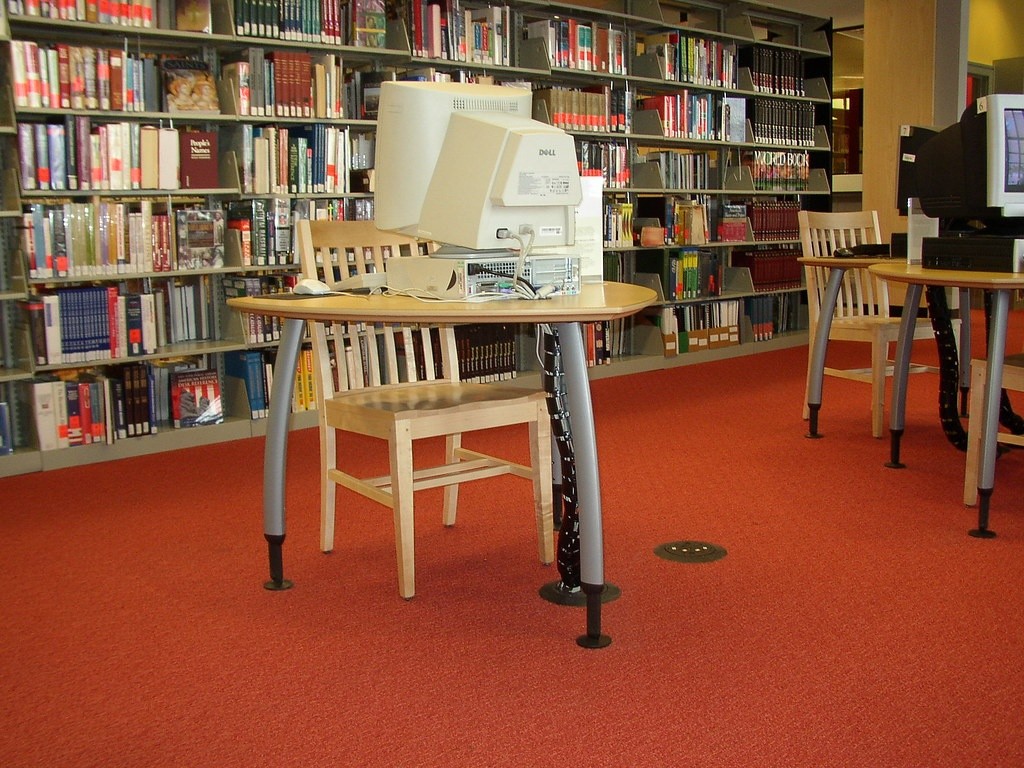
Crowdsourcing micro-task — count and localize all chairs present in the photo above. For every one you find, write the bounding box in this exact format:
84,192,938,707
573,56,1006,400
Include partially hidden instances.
296,215,558,600
962,352,1024,506
797,208,963,440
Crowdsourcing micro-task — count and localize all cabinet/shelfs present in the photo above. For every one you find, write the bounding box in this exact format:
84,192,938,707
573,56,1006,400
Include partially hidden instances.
520,0,665,382
0,0,252,472
0,1,42,478
627,1,832,376
217,0,552,442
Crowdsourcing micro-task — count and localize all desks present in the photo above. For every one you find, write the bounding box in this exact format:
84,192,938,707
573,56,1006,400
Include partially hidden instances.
867,263,1024,538
796,255,973,469
227,277,658,648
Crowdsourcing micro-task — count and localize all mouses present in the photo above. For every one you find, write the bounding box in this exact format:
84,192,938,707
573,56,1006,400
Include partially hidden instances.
293,279,330,295
834,247,854,258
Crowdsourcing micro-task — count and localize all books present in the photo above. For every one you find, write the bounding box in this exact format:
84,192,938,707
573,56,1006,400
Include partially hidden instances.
0,1,834,460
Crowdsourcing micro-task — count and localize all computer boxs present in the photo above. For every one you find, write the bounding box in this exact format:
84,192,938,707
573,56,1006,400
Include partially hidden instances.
921,236,1024,272
385,256,582,302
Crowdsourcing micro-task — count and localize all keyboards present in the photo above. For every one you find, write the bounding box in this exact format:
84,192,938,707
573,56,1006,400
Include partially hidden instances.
333,272,388,295
852,243,890,259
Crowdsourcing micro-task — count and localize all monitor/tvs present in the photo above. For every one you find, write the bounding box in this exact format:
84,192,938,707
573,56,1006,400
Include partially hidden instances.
896,125,942,217
914,94,1024,236
373,81,582,260
